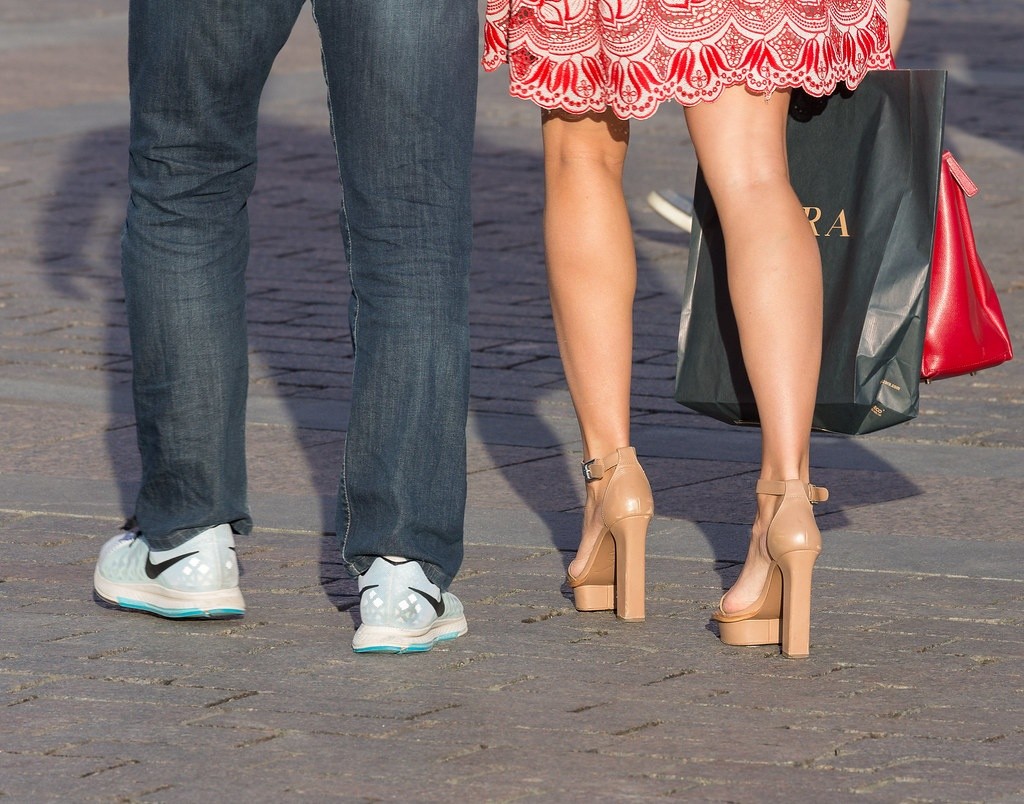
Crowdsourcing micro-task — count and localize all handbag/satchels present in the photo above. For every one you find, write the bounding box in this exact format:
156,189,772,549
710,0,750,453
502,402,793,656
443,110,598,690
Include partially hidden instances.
674,68,949,436
921,148,1014,385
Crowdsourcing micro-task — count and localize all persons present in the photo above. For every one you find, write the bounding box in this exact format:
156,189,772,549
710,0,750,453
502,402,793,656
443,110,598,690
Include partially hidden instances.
93,1,478,652
482,1,911,657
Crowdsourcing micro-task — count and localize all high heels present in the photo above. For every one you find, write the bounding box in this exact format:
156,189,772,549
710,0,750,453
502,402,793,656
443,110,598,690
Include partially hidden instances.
715,478,828,659
566,446,653,620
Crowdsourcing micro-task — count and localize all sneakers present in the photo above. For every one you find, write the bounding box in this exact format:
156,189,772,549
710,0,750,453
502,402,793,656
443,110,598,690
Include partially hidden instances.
94,522,245,618
351,554,468,654
